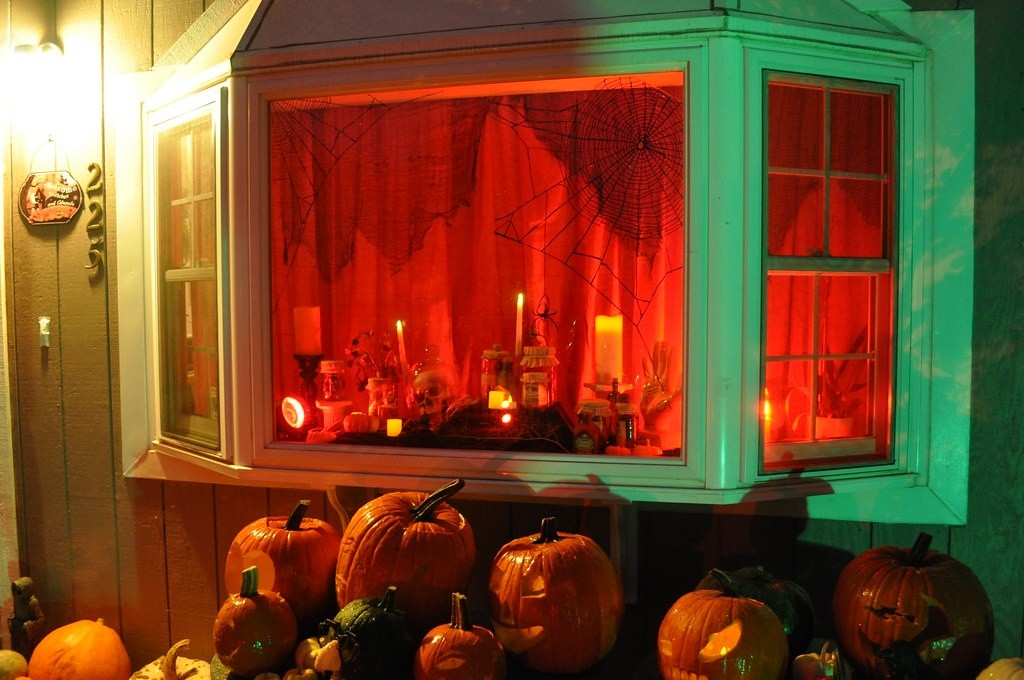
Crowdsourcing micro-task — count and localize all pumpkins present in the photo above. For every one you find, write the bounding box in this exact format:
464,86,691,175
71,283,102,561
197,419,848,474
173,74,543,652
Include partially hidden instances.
973,657,1024,680
0,617,211,680
254,635,333,680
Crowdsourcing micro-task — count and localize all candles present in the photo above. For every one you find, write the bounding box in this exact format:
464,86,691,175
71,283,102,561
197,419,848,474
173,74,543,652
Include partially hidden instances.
294,306,322,355
387,419,402,436
595,314,623,385
515,294,524,353
397,322,407,368
488,389,505,406
